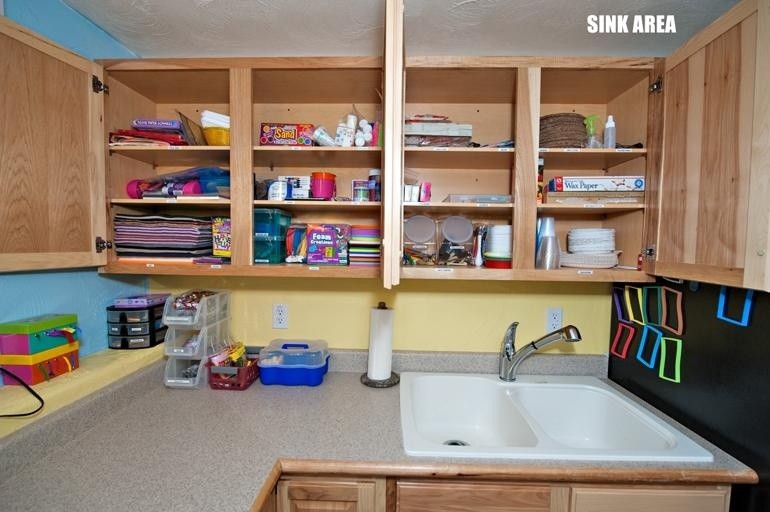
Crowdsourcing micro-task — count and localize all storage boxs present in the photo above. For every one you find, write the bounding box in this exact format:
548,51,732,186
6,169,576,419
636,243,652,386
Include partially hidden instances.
106,291,331,390
252,205,293,263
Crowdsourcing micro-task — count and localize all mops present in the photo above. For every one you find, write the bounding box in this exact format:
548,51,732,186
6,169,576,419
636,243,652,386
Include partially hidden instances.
366,307,396,381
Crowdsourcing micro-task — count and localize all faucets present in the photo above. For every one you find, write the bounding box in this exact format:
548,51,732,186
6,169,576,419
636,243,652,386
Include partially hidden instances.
498,322,583,384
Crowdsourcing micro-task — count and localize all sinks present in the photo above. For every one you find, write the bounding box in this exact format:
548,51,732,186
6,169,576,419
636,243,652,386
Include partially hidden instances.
513,373,716,462
399,371,538,458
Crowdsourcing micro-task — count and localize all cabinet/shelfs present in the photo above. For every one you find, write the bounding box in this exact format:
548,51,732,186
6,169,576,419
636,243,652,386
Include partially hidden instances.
277,475,393,511
392,477,734,512
1,1,770,293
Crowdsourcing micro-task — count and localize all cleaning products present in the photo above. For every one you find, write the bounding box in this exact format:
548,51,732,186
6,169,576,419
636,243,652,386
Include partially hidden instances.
583,113,606,149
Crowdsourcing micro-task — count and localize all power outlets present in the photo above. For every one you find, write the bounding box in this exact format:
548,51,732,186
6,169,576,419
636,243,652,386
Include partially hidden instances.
546,306,563,335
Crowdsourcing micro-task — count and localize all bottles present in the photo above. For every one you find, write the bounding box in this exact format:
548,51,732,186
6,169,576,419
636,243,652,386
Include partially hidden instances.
603,111,618,152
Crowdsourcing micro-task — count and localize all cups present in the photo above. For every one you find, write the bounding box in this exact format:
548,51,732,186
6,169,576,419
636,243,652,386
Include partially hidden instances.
308,171,337,200
